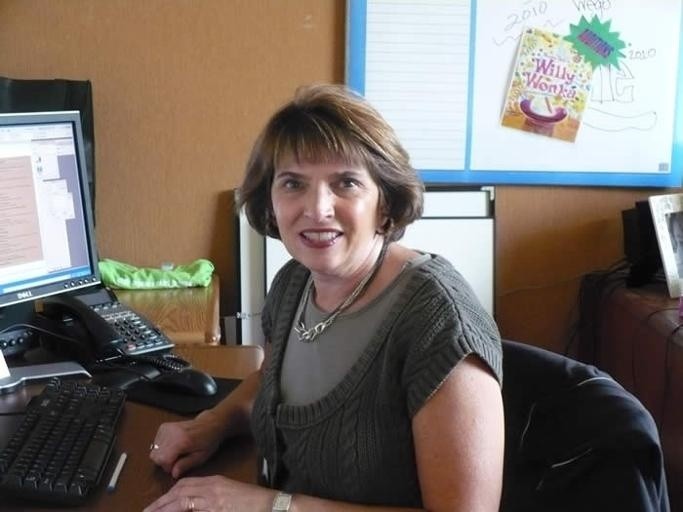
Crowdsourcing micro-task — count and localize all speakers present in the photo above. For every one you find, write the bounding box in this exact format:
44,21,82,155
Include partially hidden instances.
0,300,40,358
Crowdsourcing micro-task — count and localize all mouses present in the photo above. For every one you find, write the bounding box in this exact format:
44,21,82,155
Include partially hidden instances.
150,367,218,396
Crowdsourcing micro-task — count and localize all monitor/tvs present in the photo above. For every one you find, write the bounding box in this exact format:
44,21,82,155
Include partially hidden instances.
0,110,102,389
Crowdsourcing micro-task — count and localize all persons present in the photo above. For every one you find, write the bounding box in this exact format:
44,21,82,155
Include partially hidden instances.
142,83,506,512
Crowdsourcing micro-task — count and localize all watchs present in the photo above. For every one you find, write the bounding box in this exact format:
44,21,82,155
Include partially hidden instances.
272,491,295,511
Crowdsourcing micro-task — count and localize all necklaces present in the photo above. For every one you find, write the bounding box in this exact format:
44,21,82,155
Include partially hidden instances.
294,245,387,342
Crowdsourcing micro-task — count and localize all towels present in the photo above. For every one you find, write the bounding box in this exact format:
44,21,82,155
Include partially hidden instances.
97,254,215,290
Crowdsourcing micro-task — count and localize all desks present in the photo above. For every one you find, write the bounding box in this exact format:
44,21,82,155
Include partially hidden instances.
2,346,268,512
583,271,683,511
110,272,222,345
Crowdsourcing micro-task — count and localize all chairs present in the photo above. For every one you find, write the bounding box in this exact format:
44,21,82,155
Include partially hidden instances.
499,337,670,511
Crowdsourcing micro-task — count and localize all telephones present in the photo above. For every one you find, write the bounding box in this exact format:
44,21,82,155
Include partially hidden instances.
40,284,179,367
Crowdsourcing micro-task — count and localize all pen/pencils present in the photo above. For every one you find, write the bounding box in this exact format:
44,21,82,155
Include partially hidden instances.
108,452,128,491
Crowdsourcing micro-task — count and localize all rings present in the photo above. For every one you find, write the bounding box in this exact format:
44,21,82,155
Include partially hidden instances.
187,496,197,511
148,443,159,450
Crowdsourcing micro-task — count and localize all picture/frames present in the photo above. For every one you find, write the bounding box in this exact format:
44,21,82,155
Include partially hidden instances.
646,193,683,299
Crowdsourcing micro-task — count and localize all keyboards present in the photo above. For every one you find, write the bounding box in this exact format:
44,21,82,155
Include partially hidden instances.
0,377,128,507
